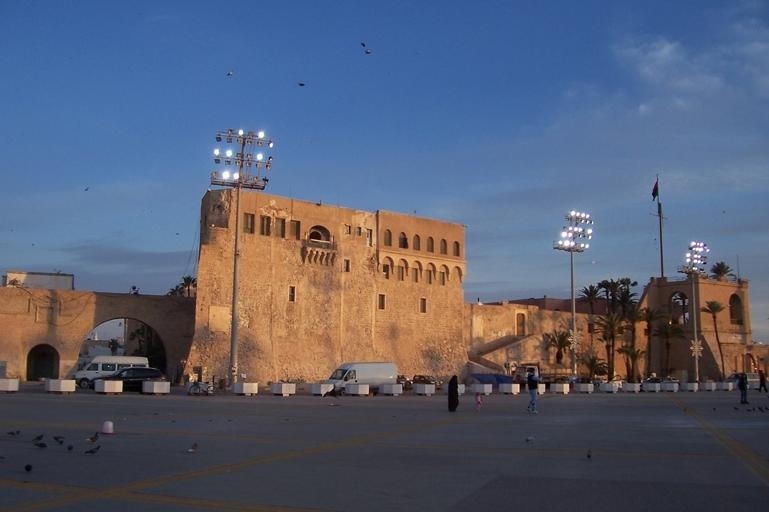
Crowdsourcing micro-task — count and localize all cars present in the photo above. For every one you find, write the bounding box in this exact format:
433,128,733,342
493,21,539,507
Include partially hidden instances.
641,376,681,384
568,375,602,384
87,368,166,391
725,372,761,390
398,374,443,391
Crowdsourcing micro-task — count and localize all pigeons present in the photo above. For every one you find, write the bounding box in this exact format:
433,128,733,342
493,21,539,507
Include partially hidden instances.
30,431,102,456
587,448,593,459
7,429,21,437
186,441,198,453
526,434,537,443
25,464,33,472
712,405,769,414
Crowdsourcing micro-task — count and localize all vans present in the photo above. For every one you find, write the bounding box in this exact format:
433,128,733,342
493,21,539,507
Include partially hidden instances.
326,361,398,396
72,355,150,389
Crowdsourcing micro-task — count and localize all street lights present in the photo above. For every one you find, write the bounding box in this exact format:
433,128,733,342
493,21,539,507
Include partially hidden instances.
211,126,274,393
677,241,711,384
176,359,187,383
552,209,594,378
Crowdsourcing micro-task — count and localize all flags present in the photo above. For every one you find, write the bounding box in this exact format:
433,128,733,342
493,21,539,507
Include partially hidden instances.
652,181,658,201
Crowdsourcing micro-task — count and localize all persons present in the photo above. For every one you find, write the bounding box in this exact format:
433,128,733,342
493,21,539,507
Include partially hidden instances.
525,369,543,415
448,374,459,411
757,369,767,393
739,371,749,405
475,391,484,410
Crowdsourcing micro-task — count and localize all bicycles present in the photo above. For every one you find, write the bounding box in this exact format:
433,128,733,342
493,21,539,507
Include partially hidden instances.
189,380,217,396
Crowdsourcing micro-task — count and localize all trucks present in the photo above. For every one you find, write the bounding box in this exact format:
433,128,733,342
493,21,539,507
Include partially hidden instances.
512,362,572,385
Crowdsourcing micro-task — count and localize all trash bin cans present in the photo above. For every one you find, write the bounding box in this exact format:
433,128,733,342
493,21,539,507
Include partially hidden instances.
219,378,225,389
